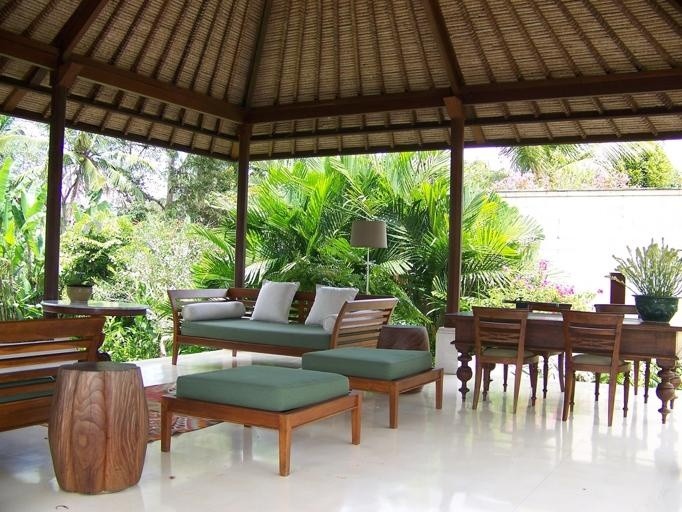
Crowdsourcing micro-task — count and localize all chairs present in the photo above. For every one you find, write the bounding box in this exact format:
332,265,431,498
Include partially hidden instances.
595,304,651,403
558,308,632,425
0,317,108,431
471,305,539,414
503,301,572,398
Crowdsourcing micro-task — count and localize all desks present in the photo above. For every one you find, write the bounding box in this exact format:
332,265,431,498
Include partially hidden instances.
39,300,147,362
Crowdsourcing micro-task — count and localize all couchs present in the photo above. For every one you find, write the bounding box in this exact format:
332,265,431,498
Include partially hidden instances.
167,288,399,365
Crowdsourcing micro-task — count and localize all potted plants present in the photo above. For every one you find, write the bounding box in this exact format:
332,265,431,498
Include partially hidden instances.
62,255,101,304
604,236,682,323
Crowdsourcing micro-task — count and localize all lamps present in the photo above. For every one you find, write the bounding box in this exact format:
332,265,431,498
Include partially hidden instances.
350,221,388,292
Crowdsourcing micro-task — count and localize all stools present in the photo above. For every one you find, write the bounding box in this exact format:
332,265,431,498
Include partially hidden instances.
161,364,362,475
302,347,444,429
48,361,149,495
377,325,430,395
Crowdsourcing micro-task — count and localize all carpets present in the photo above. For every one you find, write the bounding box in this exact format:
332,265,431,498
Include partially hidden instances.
41,382,224,443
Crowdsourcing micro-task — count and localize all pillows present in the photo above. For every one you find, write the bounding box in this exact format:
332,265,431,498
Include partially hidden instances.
304,284,359,326
250,279,301,324
181,301,245,321
322,309,374,335
